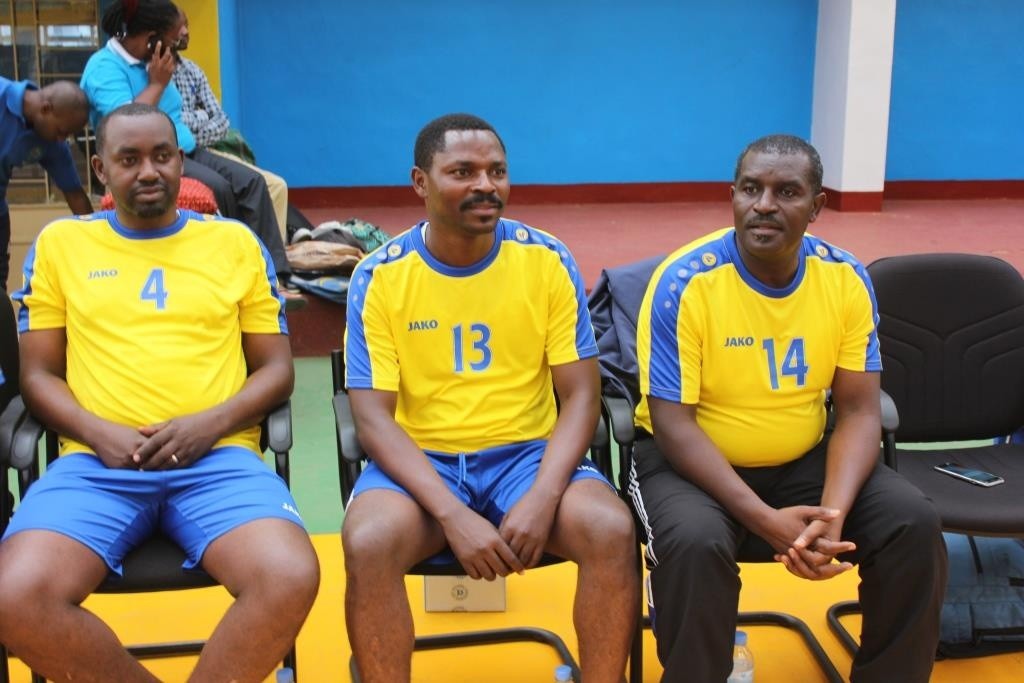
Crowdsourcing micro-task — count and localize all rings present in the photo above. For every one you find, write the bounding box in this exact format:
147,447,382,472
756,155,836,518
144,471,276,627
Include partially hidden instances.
172,454,179,464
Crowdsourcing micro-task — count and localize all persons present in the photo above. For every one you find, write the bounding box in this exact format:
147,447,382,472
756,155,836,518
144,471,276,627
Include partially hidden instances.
0,76,93,393
0,105,321,683
343,114,639,682
626,133,950,683
80,0,308,312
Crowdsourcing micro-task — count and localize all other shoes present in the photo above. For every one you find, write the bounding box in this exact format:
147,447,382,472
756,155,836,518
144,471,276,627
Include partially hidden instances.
280,289,308,310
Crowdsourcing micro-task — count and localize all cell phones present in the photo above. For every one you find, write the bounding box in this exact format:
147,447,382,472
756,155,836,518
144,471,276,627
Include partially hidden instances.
933,461,1005,487
149,32,164,57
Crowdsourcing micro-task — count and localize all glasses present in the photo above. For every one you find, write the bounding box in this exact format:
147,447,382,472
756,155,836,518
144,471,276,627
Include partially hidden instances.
160,35,181,47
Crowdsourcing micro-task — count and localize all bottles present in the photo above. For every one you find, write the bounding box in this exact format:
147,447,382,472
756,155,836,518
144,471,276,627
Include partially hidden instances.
727,631,754,683
276,668,294,683
555,665,572,683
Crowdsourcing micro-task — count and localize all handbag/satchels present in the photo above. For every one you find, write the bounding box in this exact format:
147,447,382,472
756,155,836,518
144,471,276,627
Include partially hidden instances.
284,216,395,275
935,532,1024,658
218,130,256,164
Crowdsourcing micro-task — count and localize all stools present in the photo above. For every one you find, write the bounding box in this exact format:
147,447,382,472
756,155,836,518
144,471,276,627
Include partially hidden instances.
99,176,218,214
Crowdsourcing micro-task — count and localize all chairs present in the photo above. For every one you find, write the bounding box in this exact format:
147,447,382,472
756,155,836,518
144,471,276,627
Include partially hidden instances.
329,325,616,683
599,253,844,683
826,253,1024,659
0,399,298,683
